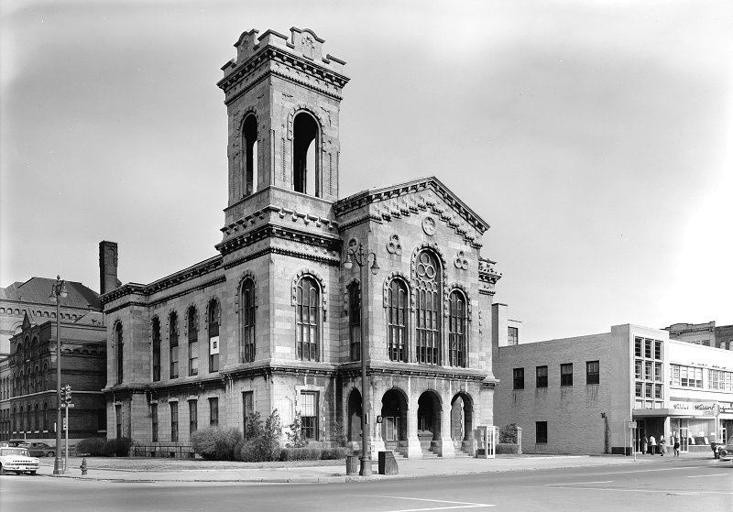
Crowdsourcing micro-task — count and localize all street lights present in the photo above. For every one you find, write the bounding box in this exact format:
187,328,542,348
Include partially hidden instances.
48,275,68,474
343,242,380,476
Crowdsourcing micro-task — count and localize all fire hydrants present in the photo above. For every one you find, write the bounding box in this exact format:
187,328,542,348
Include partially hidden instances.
80,458,87,475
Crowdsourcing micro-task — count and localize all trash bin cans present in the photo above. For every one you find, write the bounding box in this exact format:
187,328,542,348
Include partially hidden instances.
346,456,359,475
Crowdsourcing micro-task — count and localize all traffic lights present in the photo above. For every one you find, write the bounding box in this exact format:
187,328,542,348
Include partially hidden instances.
61,386,72,402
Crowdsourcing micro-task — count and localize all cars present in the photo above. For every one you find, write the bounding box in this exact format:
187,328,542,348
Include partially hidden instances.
0,441,56,476
720,436,733,461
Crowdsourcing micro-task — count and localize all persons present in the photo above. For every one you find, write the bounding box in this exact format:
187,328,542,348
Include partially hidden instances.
642,433,649,455
649,433,657,456
658,434,667,456
672,436,680,457
